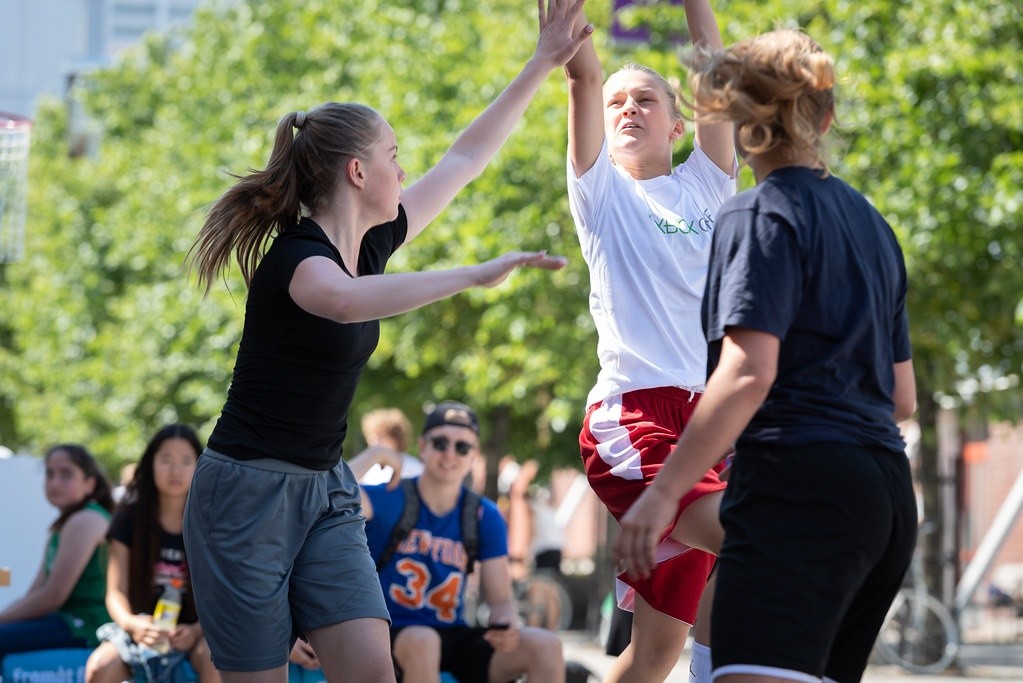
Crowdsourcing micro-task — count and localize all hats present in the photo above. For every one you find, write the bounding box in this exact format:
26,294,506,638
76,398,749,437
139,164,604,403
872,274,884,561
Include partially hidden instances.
423,400,479,436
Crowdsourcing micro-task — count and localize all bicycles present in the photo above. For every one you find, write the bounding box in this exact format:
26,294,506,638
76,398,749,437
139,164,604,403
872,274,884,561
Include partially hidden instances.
874,522,959,676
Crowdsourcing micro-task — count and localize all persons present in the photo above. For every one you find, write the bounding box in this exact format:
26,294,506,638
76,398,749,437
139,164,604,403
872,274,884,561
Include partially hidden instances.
0,400,570,683
611,30,916,682
182,0,594,682
558,1,739,683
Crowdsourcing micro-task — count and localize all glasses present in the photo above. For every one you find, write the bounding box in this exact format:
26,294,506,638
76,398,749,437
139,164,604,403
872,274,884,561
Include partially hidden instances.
429,436,477,456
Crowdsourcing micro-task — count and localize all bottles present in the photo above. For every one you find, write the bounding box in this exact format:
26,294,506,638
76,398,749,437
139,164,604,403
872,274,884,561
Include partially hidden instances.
153,578,183,637
